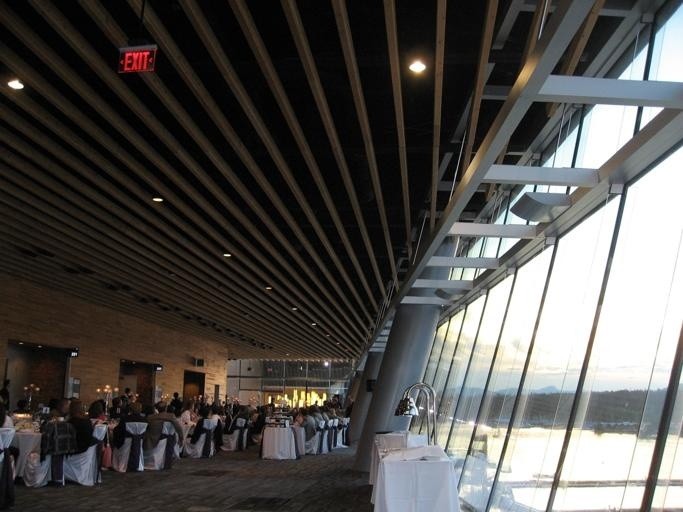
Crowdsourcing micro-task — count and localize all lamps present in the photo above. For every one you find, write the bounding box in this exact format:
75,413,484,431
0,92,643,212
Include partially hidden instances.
400,383,437,446
394,387,430,446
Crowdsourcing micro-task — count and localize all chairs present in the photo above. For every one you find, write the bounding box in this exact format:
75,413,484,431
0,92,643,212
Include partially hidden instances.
251,418,350,461
101,417,252,474
1,419,107,490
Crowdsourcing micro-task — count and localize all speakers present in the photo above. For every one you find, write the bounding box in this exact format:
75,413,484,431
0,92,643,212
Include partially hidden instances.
197,358,204,366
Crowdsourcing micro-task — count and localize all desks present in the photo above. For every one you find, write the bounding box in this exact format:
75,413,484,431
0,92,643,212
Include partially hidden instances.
370,433,460,512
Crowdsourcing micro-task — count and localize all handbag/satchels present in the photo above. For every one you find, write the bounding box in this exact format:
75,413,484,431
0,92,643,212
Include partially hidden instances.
101,445,111,468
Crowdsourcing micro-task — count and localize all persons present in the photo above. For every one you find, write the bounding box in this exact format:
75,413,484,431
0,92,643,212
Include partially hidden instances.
192,393,354,452
1,378,198,472
